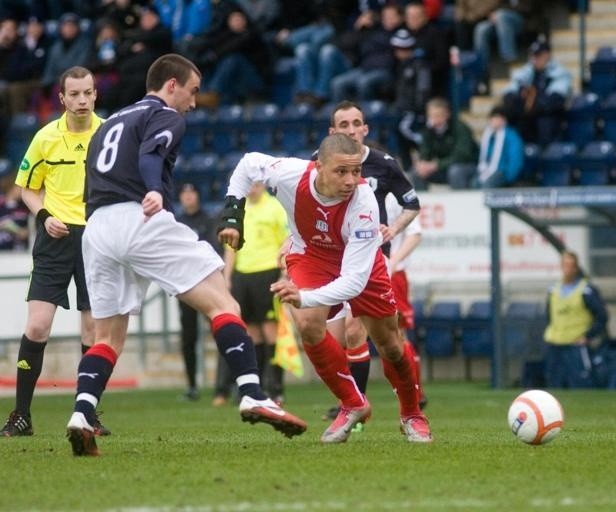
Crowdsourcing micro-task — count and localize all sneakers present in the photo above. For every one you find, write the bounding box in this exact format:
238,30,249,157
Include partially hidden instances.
419,391,428,409
399,411,434,444
320,391,371,443
238,390,308,439
0,410,34,438
176,388,286,407
65,412,111,457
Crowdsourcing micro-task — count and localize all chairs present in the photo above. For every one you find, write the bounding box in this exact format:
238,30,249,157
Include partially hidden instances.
366,299,547,383
521,49,615,186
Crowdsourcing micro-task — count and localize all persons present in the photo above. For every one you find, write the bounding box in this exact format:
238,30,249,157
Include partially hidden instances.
230,181,292,410
1,0,575,189
171,179,229,410
216,133,432,446
63,53,308,456
309,98,428,421
542,251,608,387
0,67,111,440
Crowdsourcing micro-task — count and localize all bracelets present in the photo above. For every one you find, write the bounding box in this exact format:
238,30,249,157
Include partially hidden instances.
35,208,52,227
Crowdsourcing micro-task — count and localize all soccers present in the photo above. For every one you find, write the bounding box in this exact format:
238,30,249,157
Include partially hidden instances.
508,390,563,444
